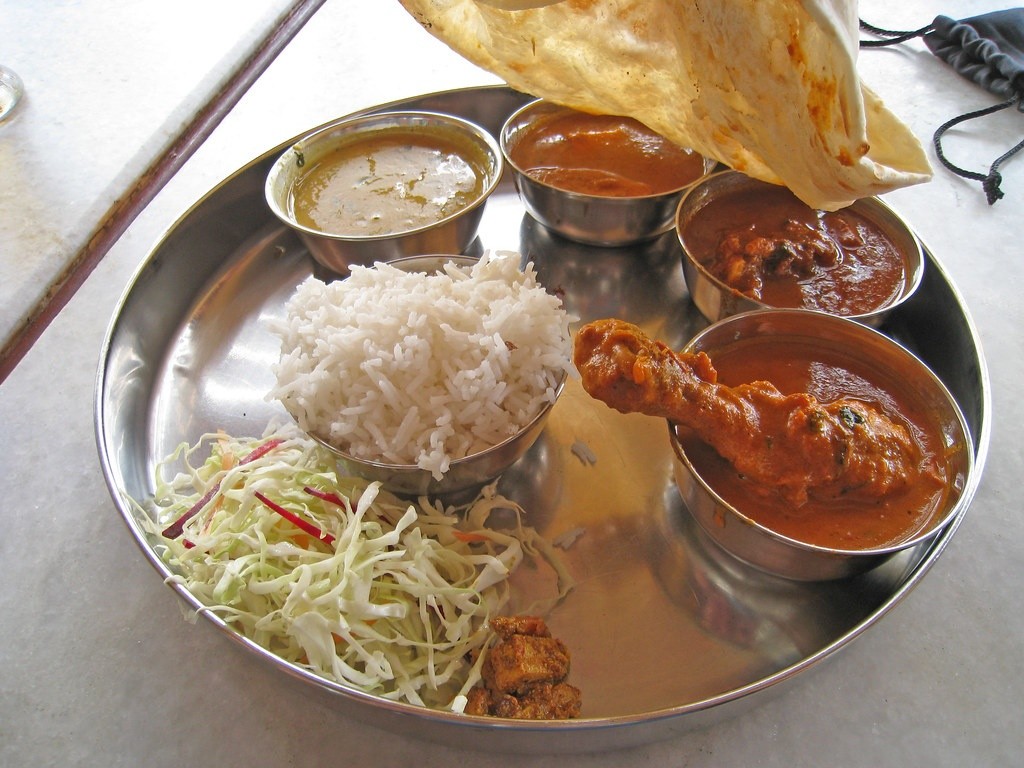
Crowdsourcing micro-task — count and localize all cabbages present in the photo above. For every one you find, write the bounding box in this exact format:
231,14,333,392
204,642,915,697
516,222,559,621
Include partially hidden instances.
117,422,576,715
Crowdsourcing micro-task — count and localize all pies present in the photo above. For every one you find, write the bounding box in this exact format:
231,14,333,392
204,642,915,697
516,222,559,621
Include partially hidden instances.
402,0,935,211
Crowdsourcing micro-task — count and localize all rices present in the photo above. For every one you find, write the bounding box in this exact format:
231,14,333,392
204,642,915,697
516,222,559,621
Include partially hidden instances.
263,250,597,552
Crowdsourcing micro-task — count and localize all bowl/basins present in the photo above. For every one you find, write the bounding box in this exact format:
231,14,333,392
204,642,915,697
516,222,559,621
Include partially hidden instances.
265,111,504,276
666,308,976,581
499,98,718,247
279,253,572,495
674,169,925,333
